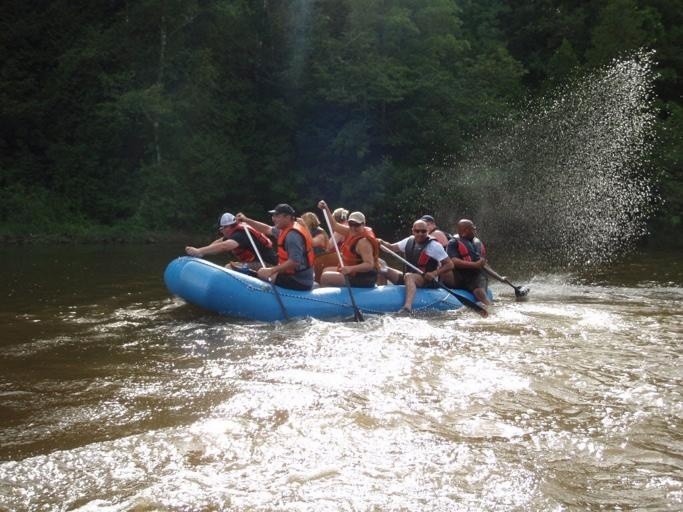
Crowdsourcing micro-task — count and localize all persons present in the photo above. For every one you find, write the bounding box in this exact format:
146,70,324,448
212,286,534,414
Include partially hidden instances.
316,200,376,288
183,212,277,269
295,210,329,256
375,219,455,315
312,206,351,280
420,214,449,251
233,202,313,292
437,218,491,306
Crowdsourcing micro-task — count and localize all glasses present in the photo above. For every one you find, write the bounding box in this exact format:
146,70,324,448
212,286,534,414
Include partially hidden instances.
348,223,364,228
413,228,428,234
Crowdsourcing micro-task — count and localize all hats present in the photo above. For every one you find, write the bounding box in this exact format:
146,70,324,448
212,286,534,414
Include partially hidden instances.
420,214,435,223
216,211,237,228
346,210,367,226
266,202,297,217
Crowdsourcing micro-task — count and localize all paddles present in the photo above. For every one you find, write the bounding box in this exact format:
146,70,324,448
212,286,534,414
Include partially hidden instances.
321,208,366,322
504,278,529,298
379,243,488,320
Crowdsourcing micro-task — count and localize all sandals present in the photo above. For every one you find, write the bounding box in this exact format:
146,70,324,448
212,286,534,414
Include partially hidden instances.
396,306,413,318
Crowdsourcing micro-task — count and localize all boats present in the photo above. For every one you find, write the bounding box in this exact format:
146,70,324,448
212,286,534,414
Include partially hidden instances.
161,254,494,325
